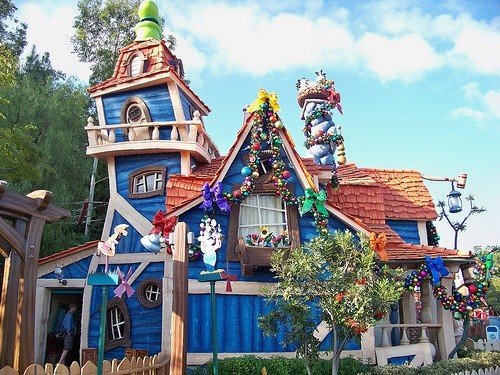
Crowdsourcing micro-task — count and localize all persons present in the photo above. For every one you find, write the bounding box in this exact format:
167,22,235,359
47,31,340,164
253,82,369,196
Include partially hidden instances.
55,302,79,365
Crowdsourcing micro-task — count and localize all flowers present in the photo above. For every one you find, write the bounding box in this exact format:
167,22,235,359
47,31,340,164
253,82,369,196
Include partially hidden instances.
245,226,290,248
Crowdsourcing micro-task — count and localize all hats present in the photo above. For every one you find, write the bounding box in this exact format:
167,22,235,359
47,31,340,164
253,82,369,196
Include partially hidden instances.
68,303,78,308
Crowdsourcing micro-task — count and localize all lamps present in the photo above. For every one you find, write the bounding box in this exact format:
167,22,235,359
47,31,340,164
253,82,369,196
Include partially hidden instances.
447,180,462,213
54,264,67,286
140,233,175,253
